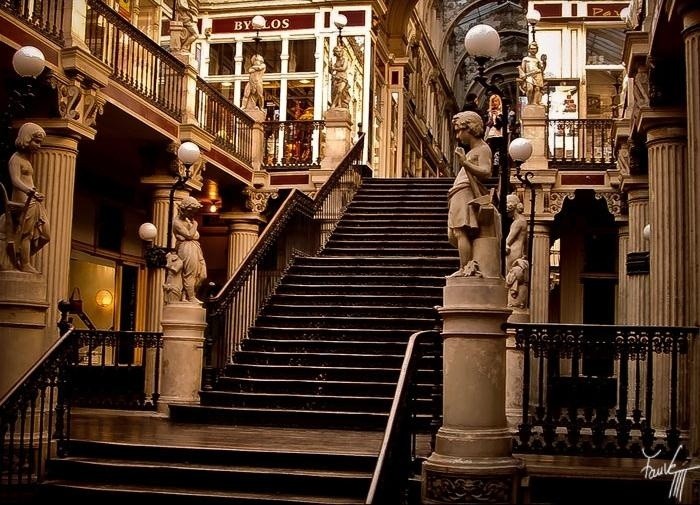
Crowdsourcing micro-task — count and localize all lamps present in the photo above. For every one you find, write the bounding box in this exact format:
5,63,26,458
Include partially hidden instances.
138,142,220,269
334,14,348,46
641,224,650,265
0,46,45,201
252,15,266,57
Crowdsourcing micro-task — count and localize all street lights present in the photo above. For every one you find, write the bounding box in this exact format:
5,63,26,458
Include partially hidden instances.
248,16,264,57
335,14,347,48
5,46,45,118
138,141,201,270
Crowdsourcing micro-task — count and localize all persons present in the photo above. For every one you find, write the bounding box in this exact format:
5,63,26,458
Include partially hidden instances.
328,46,349,109
483,92,508,177
462,93,478,111
522,41,543,104
172,196,208,304
8,123,51,274
445,110,492,277
175,0,199,52
240,54,267,113
506,194,528,276
